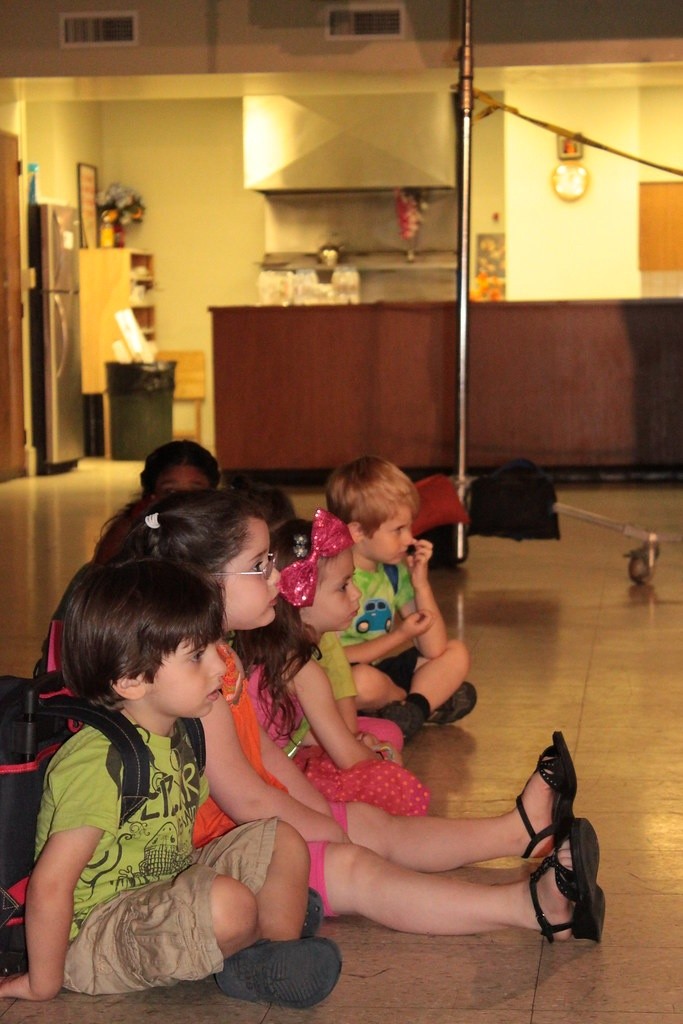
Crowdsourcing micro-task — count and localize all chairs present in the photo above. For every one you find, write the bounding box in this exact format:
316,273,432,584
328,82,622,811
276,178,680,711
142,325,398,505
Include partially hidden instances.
158,351,206,443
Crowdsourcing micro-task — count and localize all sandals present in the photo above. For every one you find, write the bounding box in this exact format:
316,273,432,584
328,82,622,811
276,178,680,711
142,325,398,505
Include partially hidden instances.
300,886,326,941
516,731,576,859
529,818,604,943
214,935,343,1009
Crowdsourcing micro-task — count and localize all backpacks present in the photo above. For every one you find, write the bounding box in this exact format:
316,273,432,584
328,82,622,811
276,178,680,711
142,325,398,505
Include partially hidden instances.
0,671,206,977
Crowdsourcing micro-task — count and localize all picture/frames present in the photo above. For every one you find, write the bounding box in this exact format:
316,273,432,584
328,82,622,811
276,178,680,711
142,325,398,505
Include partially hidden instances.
559,133,584,160
77,162,100,249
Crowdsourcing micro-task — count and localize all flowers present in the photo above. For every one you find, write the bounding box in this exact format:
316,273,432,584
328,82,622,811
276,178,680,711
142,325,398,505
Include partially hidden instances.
94,179,146,226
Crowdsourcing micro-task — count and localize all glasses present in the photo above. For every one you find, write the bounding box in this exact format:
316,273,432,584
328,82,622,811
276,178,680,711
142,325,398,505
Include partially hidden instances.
207,549,279,580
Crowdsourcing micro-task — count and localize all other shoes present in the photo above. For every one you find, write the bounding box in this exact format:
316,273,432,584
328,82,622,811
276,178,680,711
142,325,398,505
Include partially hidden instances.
426,681,477,724
374,700,423,740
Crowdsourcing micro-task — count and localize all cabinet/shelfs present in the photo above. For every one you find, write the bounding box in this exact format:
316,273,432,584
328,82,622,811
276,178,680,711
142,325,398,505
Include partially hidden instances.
78,250,154,395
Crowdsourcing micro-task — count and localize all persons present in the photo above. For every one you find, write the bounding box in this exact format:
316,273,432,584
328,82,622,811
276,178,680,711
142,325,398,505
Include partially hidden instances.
0,557,341,1007
102,438,605,942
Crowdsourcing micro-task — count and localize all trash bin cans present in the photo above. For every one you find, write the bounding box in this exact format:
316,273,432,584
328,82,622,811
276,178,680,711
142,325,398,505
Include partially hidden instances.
105,361,177,460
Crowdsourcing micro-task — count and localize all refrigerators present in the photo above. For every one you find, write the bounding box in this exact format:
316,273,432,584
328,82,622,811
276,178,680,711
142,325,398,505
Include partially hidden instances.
25,200,84,477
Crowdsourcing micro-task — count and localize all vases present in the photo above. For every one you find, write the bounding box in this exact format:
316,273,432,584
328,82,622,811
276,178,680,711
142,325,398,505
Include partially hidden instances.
112,222,126,248
